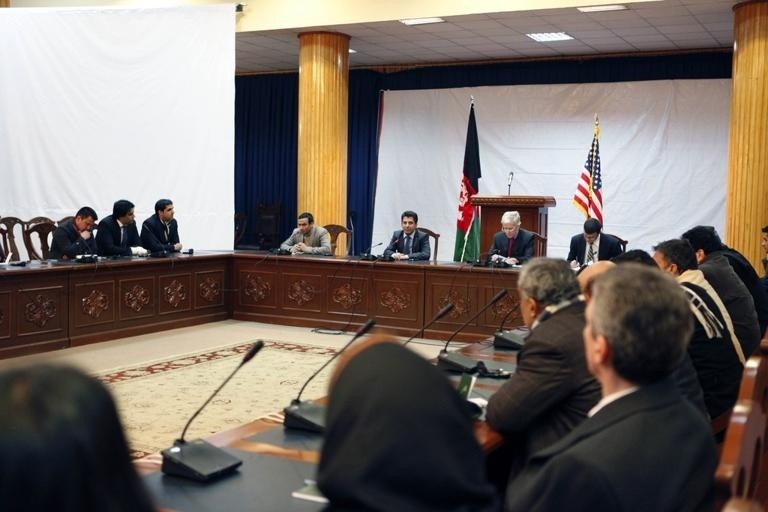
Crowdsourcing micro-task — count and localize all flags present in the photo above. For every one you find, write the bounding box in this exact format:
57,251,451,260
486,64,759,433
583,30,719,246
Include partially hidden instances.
573,132,603,230
452,103,481,262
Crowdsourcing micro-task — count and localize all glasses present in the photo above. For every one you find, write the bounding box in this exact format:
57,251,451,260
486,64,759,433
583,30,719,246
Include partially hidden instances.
501,226,515,233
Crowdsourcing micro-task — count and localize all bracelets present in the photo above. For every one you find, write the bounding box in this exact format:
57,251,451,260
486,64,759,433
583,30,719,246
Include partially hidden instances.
289,247,292,252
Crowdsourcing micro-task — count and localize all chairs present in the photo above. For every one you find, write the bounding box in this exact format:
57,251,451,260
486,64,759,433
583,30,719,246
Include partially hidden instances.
532,232,547,257
611,235,628,252
0,216,97,261
418,227,440,260
322,224,352,256
715,330,768,512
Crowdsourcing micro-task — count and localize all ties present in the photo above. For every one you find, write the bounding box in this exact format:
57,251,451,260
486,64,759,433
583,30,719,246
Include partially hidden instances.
121,225,129,247
403,235,411,256
507,238,515,256
587,242,594,262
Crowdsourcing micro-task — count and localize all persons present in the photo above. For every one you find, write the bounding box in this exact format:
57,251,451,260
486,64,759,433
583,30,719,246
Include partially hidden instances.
316,334,495,512
280,213,332,256
567,219,767,425
383,211,430,261
506,260,719,511
488,211,535,265
0,364,159,512
486,256,601,472
95,200,148,257
139,199,182,253
49,207,98,260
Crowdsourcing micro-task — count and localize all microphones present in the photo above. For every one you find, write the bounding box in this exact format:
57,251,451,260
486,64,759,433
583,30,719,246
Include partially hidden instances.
402,303,453,347
143,223,168,258
437,289,509,374
160,340,264,483
382,236,407,262
585,252,597,263
361,243,383,261
494,303,526,349
277,231,299,255
283,318,375,433
495,249,510,268
508,172,513,187
74,231,98,263
